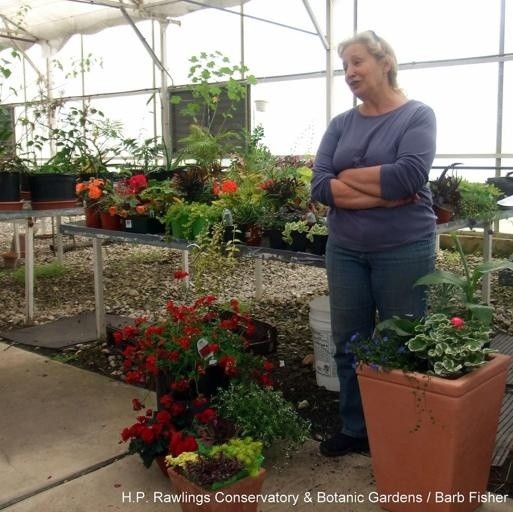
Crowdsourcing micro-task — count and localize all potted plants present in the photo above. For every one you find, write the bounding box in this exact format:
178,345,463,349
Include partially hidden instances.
163,202,191,239
261,203,284,249
145,138,190,183
305,222,328,255
163,437,269,511
244,197,261,246
30,156,66,210
61,163,81,211
213,198,243,246
97,195,121,231
209,381,312,473
115,395,223,468
16,161,36,200
280,216,309,252
0,148,24,214
430,162,462,224
75,178,104,200
74,153,114,192
149,203,167,235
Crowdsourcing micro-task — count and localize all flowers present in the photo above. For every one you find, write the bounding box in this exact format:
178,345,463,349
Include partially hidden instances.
115,172,150,196
208,175,239,198
111,271,277,390
115,199,149,217
343,234,512,380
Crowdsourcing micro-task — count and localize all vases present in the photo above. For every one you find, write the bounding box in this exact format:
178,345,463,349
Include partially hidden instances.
151,368,230,432
120,215,152,235
80,199,99,232
355,353,512,512
145,452,168,473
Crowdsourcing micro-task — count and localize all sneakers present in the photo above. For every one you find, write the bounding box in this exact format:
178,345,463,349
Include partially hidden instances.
318,431,370,462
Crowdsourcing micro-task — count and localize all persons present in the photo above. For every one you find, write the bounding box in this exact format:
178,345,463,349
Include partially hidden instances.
311,31,438,457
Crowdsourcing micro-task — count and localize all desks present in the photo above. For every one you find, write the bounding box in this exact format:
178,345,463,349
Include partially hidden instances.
0,194,200,326
54,205,513,344
